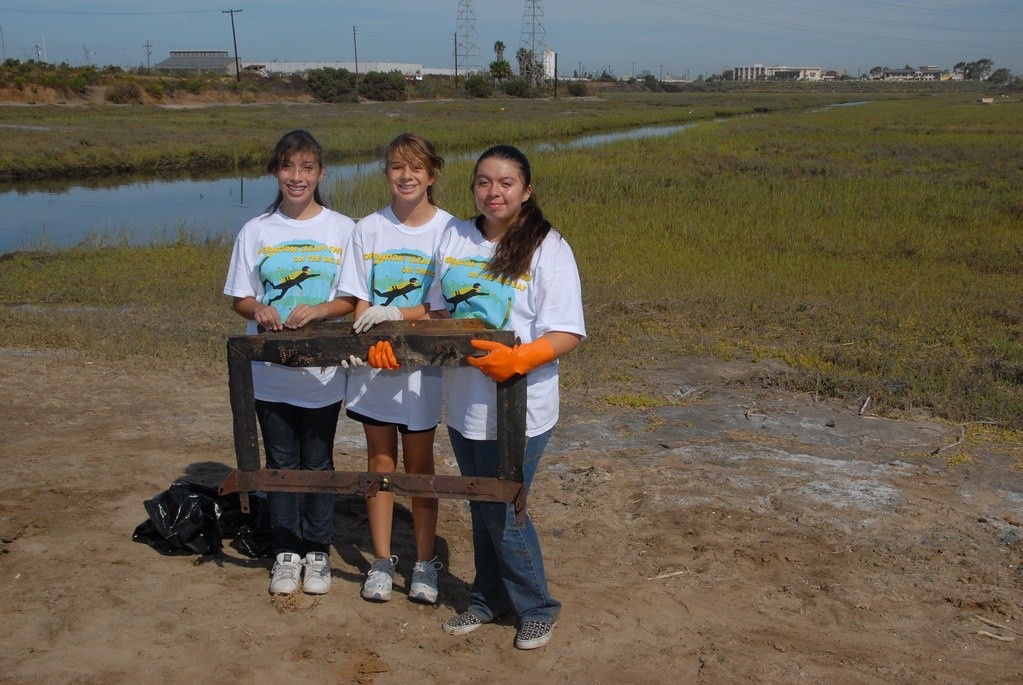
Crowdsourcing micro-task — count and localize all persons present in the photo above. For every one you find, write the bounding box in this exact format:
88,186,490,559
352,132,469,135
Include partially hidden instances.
338,136,464,603
222,129,355,595
366,143,586,648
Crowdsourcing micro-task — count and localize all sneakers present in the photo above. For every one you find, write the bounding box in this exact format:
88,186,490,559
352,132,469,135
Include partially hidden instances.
269,552,307,595
362,554,399,601
302,553,333,594
408,554,444,603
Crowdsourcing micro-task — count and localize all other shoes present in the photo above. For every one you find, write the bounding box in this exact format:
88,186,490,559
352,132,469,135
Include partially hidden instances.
515,619,558,649
442,609,483,635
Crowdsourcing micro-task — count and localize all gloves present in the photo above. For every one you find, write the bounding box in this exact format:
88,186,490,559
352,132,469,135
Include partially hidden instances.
354,304,402,335
369,339,399,372
467,337,554,383
341,356,367,375
